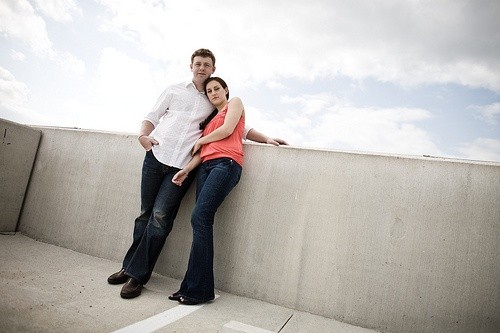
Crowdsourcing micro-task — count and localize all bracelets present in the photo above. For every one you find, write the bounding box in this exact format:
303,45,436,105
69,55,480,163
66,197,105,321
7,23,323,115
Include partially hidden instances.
138,135,144,140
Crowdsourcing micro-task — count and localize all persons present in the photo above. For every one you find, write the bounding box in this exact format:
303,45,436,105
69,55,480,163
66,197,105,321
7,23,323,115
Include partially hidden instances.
170,76,245,304
107,48,288,299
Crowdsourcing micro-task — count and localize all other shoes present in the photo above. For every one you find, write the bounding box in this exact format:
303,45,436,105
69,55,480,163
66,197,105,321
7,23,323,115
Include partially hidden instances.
168,289,215,305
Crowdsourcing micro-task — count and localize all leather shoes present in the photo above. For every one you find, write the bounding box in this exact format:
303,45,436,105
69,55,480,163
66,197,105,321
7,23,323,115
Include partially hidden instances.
120,277,144,299
108,268,129,285
